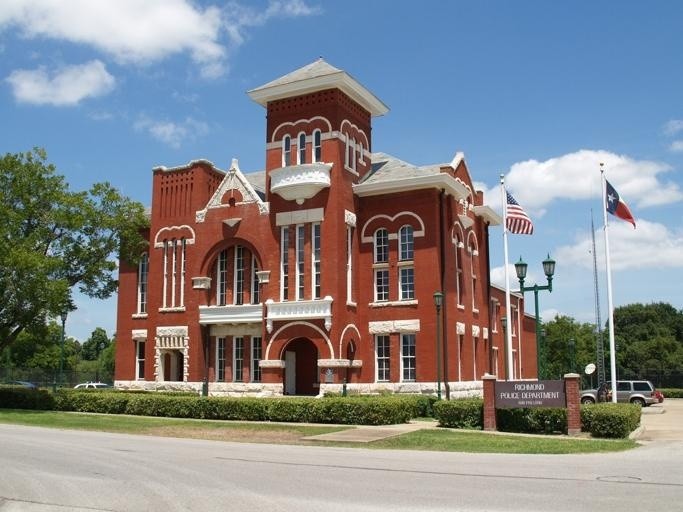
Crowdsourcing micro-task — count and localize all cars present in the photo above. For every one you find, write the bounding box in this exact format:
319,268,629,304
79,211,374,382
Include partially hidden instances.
4,380,38,391
655,389,664,402
75,382,112,389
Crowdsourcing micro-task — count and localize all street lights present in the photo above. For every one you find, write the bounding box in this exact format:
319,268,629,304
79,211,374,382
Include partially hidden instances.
499,316,509,382
95,341,105,382
430,289,443,406
515,252,559,381
56,309,70,393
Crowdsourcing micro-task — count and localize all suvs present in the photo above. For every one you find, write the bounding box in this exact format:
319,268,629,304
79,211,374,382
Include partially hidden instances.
580,379,660,407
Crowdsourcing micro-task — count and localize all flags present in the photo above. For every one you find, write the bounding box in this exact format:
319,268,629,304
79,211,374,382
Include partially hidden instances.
605,178,636,230
504,190,533,235
